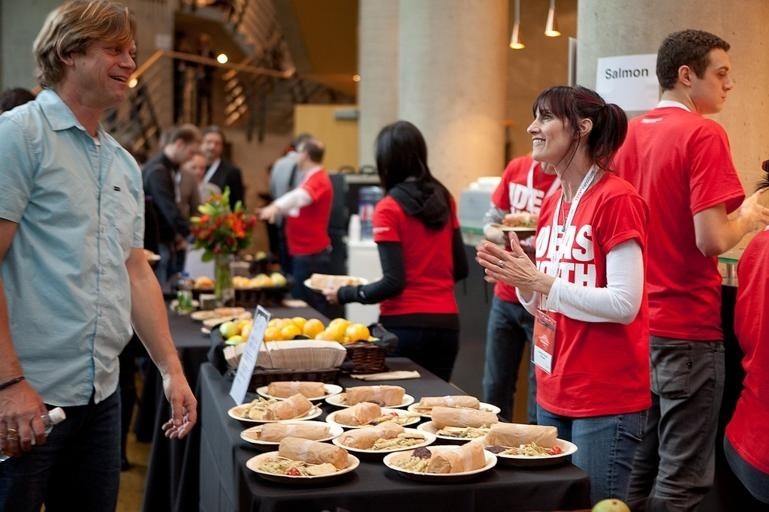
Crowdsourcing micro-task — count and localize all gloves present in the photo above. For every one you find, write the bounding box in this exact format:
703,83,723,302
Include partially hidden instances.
351,370,422,381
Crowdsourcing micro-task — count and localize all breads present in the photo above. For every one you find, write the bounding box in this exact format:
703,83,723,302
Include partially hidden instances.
277,436,350,472
347,424,410,446
345,385,407,406
262,420,328,439
269,380,326,399
306,274,360,292
267,393,315,418
430,408,498,429
421,395,481,410
485,421,559,449
433,445,485,473
335,402,384,425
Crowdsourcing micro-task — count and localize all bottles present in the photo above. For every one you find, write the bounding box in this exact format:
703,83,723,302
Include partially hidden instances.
174,271,191,316
0,407,66,463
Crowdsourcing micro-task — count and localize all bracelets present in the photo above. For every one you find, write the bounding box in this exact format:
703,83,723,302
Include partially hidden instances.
0,375,26,392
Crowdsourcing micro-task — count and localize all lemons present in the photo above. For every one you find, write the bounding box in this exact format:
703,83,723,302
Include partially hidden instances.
220,317,253,345
263,316,379,344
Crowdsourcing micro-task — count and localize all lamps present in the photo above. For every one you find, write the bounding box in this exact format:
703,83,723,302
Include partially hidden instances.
544,1,561,37
509,0,525,50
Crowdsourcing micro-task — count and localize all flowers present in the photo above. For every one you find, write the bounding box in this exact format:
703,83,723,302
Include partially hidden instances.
190,186,255,255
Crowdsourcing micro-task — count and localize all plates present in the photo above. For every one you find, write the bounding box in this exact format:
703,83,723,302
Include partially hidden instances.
226,402,323,424
417,419,488,442
499,223,537,233
255,382,343,401
244,450,360,479
240,419,343,445
381,445,498,477
325,408,419,429
325,389,414,409
471,434,578,461
407,399,501,420
305,275,368,292
331,427,436,454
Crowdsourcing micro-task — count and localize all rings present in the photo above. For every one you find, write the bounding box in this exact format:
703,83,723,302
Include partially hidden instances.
496,259,504,267
6,427,18,440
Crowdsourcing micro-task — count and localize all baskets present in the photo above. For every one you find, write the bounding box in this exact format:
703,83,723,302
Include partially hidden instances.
232,367,340,391
340,344,386,374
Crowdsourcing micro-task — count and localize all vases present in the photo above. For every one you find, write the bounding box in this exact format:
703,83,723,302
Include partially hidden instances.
214,253,233,307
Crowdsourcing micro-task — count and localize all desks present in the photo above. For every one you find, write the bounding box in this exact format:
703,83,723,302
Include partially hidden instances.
142,287,404,512
201,356,591,510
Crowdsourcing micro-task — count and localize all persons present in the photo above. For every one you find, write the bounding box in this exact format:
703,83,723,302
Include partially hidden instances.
0,86,37,113
158,127,202,223
253,138,335,309
200,130,246,214
607,26,768,512
0,0,200,511
322,119,470,385
179,151,222,206
141,123,200,291
475,84,654,511
482,153,563,427
723,158,769,512
269,132,312,278
